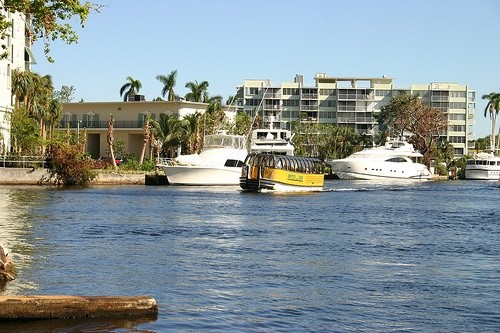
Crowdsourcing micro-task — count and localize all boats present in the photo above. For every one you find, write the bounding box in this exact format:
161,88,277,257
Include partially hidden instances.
464,152,499,180
240,152,324,192
157,115,296,186
328,135,448,181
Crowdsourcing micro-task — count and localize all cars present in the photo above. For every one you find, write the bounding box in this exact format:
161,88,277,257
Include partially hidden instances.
98,156,123,166
